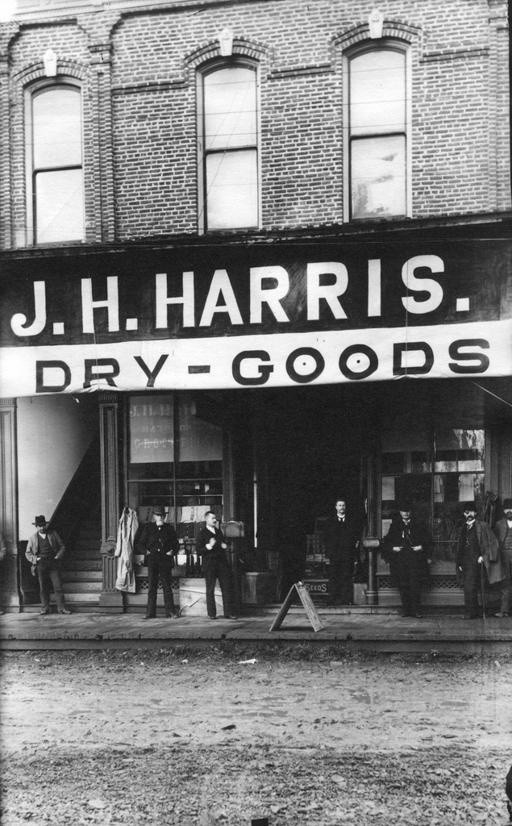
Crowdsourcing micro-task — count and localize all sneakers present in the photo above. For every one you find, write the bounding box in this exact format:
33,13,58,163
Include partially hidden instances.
142,611,178,619
40,610,48,615
58,609,72,614
208,614,239,619
459,607,512,619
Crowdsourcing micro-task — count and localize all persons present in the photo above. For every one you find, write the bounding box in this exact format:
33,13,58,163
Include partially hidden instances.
134,505,182,620
320,498,359,609
23,515,73,614
379,499,429,618
492,499,512,618
453,500,506,616
193,509,238,620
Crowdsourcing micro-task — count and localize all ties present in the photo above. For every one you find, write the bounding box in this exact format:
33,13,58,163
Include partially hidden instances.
338,517,346,528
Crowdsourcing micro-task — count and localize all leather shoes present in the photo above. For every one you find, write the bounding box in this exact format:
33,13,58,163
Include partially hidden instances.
401,608,423,619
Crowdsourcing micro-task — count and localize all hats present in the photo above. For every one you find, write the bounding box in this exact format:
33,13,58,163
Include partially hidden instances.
151,505,169,515
32,515,50,525
